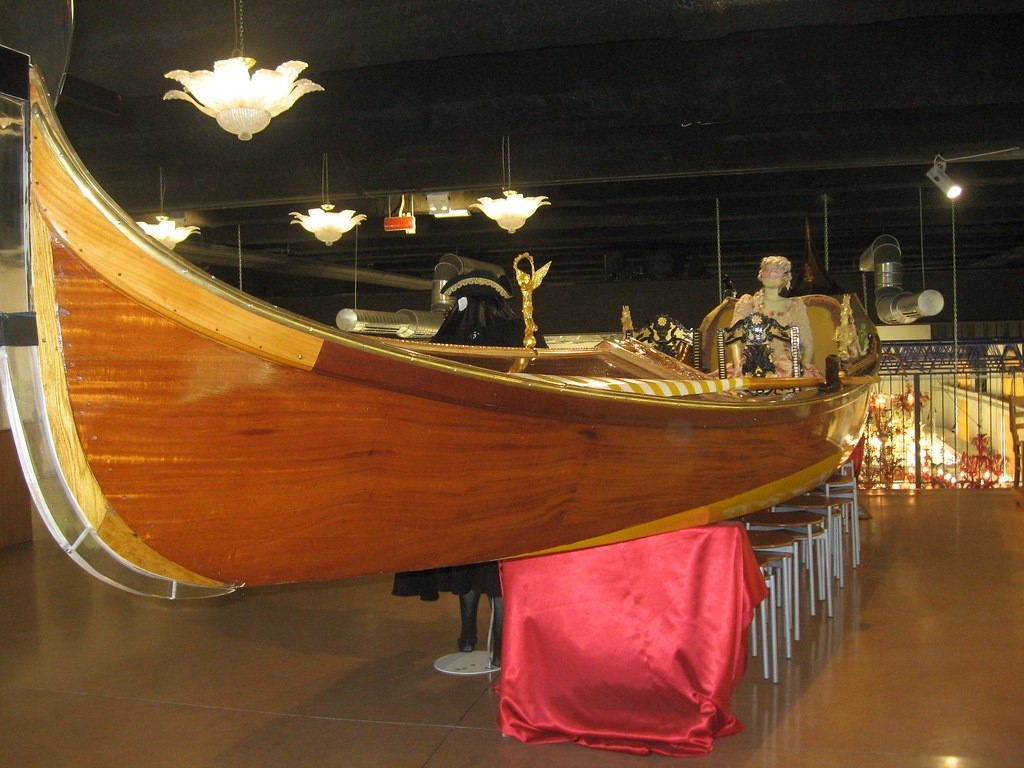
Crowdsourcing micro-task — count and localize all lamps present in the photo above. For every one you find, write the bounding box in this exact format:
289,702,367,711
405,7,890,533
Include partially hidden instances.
288,152,367,245
926,146,1020,200
162,0,327,141
468,136,553,234
135,166,202,251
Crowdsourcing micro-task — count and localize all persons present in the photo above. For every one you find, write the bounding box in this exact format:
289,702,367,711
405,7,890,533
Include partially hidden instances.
729,255,815,379
833,293,860,359
513,252,552,335
393,267,548,668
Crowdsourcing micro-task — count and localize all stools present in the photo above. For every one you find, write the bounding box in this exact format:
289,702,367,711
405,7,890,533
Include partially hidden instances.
740,460,862,684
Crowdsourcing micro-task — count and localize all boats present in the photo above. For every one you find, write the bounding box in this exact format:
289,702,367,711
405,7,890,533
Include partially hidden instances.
1,1,886,604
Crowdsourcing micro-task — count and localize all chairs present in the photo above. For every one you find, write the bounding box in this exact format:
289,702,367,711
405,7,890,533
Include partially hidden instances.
1009,395,1024,489
